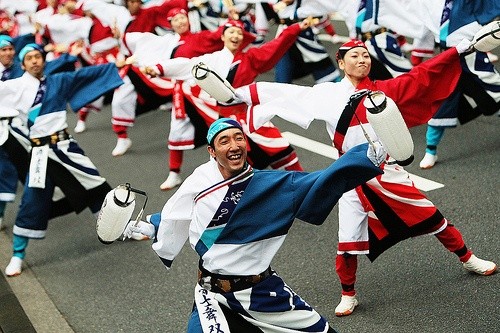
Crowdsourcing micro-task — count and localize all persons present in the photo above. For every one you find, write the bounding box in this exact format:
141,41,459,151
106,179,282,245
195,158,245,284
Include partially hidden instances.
124,120,387,333
218,39,498,316
0,0,500,275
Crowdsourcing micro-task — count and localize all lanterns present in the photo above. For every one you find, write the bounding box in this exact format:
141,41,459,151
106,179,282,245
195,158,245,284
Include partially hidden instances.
96,184,138,244
191,64,236,105
362,91,415,168
470,21,500,52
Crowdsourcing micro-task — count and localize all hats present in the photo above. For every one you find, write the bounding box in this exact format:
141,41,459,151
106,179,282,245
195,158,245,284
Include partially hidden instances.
338,41,366,57
167,8,187,23
0,34,14,49
206,118,242,144
223,21,244,32
18,43,44,61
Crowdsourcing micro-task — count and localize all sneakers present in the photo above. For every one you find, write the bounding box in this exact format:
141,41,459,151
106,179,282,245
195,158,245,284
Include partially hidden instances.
420,152,438,169
160,172,181,190
462,255,497,275
335,293,356,316
111,138,132,156
5,256,21,276
75,120,86,134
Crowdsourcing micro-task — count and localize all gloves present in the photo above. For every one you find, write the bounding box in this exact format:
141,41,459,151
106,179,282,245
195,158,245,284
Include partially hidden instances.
367,142,387,166
122,221,156,242
456,38,474,54
218,98,240,105
